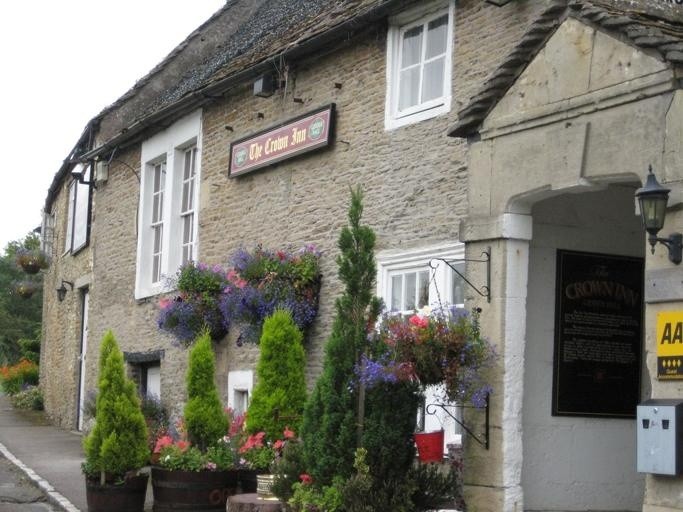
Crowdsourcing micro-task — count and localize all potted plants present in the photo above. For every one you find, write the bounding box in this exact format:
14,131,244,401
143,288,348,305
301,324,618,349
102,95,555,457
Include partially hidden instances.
80,328,151,511
152,327,229,509
231,307,307,493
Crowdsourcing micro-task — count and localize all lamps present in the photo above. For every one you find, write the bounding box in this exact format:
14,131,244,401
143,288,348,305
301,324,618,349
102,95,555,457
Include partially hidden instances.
56,277,74,302
632,162,683,267
251,78,285,98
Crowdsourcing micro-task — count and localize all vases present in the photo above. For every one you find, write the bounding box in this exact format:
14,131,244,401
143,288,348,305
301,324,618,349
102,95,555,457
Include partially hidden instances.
22,265,39,274
20,291,32,299
413,427,446,462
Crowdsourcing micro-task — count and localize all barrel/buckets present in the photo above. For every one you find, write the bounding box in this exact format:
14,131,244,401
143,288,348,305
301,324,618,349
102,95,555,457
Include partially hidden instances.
86,466,272,511
411,414,444,462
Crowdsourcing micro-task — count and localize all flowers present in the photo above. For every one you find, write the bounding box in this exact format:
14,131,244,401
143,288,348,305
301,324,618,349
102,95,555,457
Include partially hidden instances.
347,304,492,410
10,278,41,295
154,242,323,347
151,433,231,470
232,409,297,465
14,246,49,269
